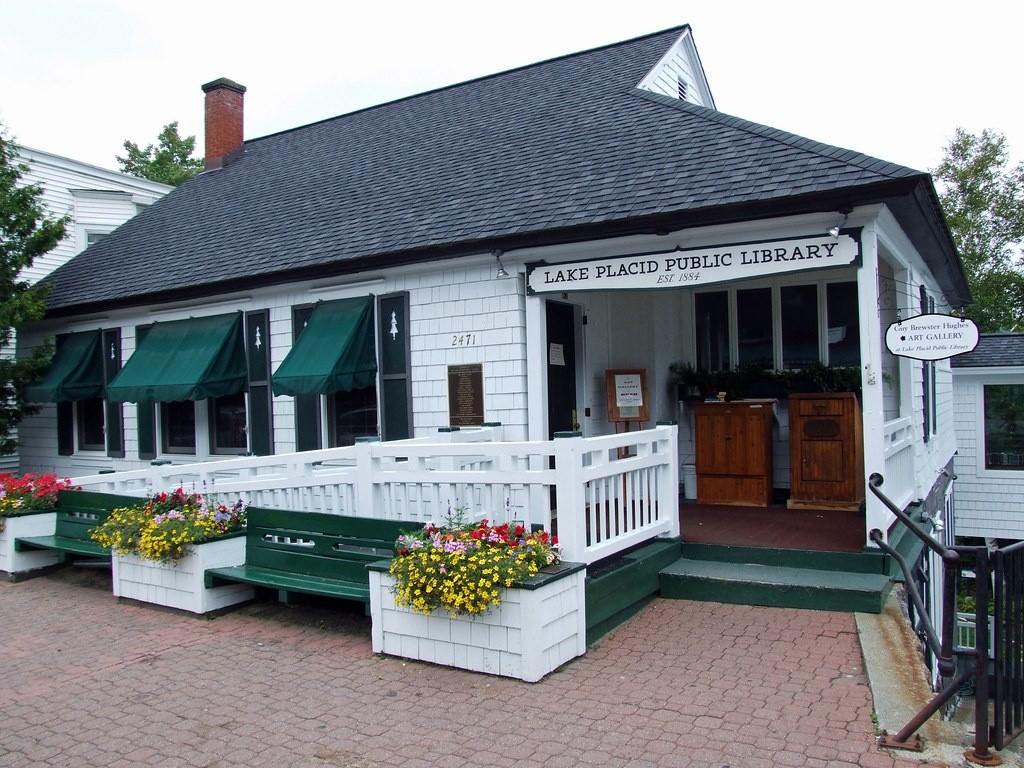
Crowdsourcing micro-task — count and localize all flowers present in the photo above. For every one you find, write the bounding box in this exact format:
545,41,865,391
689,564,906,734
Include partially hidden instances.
87,487,253,567
389,496,565,621
0,463,81,514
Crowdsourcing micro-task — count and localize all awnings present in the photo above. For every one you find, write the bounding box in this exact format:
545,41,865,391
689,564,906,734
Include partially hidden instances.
271,294,378,397
18,329,106,404
106,311,248,403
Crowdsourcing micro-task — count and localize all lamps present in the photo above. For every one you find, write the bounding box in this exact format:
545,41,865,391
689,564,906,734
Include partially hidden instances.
491,249,509,280
921,513,945,534
826,207,854,240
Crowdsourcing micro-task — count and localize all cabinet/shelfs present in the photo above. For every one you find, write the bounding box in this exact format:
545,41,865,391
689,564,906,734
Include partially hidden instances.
786,393,866,511
694,402,774,507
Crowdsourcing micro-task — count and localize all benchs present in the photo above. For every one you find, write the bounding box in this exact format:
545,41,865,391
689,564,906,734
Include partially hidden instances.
203,506,427,616
15,489,152,571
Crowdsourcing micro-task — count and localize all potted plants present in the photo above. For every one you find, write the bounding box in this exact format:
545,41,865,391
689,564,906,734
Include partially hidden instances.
666,356,862,405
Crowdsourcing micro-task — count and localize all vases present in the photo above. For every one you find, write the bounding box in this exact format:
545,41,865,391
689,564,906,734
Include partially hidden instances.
112,529,256,620
363,558,588,684
0,507,68,582
